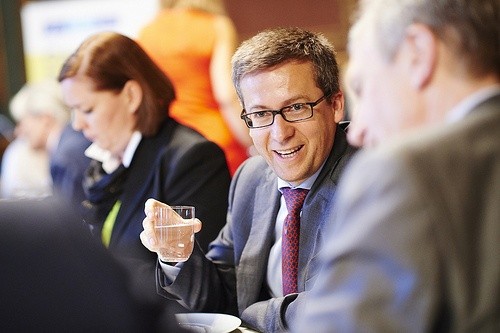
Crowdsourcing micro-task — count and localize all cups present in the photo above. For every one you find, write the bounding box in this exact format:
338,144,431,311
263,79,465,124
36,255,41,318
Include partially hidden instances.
154,206,196,262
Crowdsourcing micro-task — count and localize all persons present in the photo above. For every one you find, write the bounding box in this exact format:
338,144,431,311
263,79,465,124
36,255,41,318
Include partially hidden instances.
58,32,231,333
140,26,358,333
0,196,180,333
134,0,254,178
10,79,93,211
289,0,500,332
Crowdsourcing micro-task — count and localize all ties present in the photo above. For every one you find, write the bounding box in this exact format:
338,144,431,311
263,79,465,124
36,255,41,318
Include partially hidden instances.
282,187,311,297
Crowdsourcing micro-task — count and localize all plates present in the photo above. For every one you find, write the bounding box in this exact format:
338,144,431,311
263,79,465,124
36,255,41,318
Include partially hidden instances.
175,313,242,333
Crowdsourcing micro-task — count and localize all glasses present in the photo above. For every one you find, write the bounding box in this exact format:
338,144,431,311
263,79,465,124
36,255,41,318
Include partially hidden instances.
240,90,330,129
